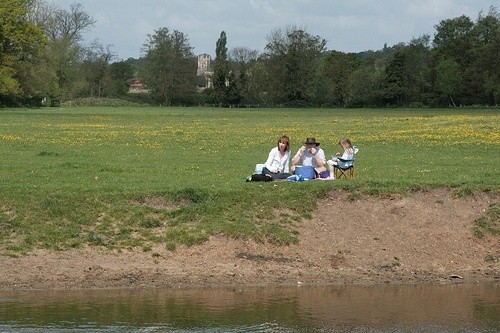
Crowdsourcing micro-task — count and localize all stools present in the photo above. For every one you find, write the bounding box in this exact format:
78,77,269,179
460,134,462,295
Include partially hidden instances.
334,157,354,179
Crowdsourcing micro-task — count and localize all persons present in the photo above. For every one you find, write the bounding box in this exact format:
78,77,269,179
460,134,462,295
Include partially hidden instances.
290,138,327,179
255,135,291,180
327,138,358,179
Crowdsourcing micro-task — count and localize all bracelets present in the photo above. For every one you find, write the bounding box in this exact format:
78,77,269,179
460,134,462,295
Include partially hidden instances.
298,151,302,158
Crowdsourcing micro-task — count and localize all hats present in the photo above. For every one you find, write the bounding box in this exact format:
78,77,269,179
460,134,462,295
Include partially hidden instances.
304,138,320,146
